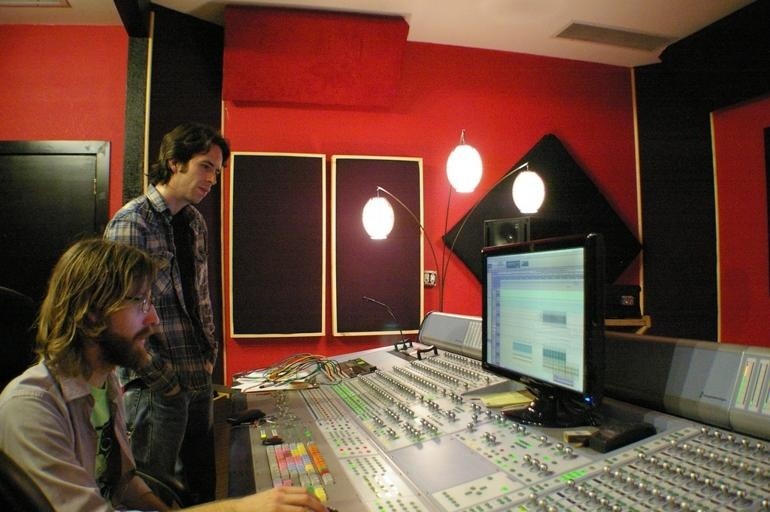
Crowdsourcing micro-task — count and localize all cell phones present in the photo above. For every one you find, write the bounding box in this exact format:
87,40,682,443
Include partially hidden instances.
227,408,266,425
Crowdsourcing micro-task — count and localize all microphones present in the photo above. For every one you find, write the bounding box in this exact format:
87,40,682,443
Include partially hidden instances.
362,295,407,350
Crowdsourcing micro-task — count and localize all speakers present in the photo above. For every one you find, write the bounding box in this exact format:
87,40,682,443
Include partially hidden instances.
483,216,570,247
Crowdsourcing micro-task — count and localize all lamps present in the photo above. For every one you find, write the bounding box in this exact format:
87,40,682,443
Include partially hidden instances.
362,129,546,314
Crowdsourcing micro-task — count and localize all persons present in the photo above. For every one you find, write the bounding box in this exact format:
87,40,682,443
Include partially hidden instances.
0,236,330,512
102,119,231,508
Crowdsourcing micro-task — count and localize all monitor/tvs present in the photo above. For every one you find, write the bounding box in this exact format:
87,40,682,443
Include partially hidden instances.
481,234,615,428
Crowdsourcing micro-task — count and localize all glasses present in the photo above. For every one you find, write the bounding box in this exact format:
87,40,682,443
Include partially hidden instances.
117,292,157,314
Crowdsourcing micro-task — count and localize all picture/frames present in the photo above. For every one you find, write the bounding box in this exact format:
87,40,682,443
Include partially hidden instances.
330,155,424,338
230,151,327,338
